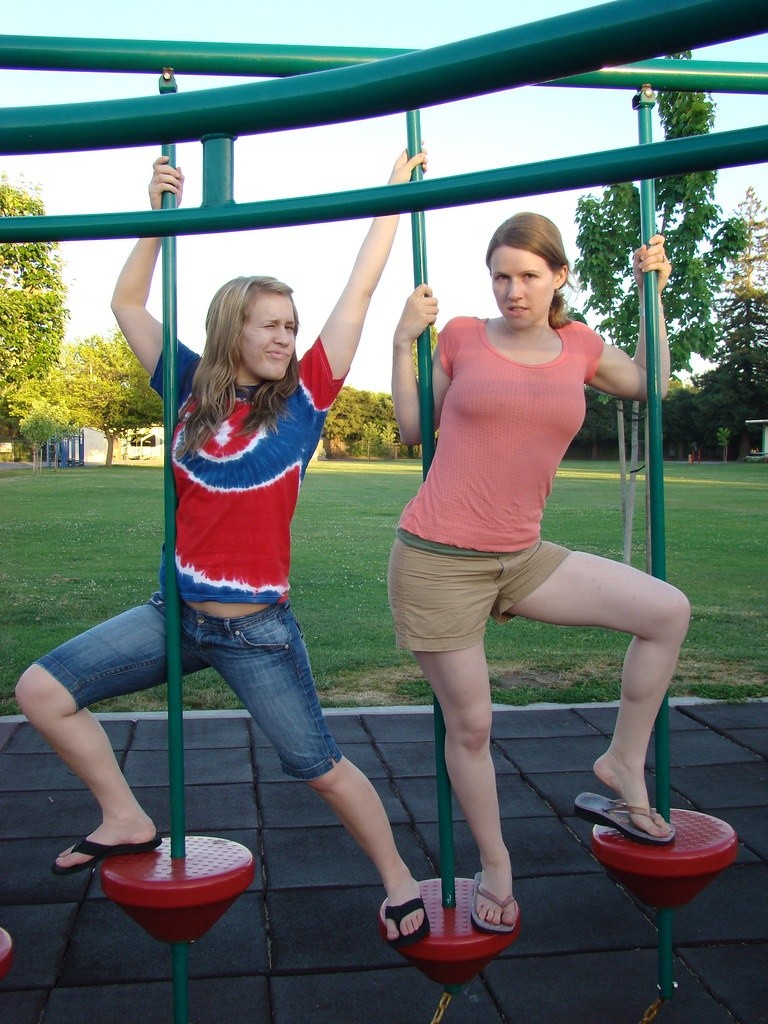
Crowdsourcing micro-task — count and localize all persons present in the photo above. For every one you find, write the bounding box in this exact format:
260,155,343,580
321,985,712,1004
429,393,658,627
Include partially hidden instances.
15,141,428,947
388,212,691,935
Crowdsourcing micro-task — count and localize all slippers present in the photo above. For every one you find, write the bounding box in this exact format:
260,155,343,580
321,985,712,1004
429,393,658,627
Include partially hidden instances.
574,792,676,844
52,832,162,876
385,893,430,950
470,872,516,933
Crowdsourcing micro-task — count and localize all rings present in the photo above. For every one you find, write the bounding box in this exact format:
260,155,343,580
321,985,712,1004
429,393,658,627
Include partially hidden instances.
663,256,666,263
